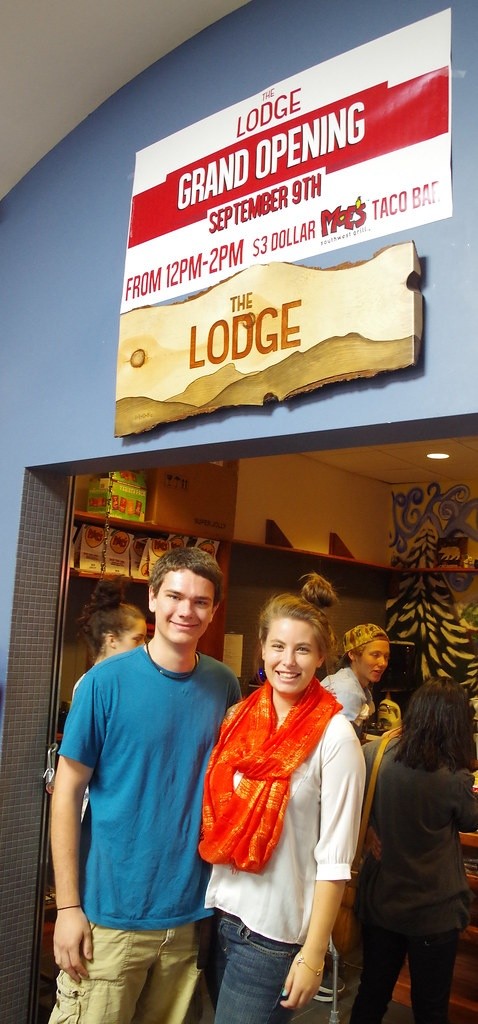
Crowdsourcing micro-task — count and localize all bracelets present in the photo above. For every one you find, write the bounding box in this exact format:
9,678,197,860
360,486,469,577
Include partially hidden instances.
296,950,326,976
56,905,81,911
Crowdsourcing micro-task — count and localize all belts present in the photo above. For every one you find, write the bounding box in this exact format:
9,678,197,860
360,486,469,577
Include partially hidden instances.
196,918,211,970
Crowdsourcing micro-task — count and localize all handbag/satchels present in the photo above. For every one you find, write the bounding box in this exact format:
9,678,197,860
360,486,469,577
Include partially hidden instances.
332,869,363,955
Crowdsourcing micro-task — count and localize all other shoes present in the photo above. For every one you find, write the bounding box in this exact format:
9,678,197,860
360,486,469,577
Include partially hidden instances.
319,970,346,994
312,991,340,1002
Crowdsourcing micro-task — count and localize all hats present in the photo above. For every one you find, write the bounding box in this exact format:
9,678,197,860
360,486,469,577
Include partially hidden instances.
336,623,390,672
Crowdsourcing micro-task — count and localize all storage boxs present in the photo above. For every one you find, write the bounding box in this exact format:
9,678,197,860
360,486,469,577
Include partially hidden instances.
145,458,240,538
87,470,147,523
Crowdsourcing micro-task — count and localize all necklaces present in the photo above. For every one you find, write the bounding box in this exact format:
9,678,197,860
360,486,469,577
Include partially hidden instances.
145,641,198,680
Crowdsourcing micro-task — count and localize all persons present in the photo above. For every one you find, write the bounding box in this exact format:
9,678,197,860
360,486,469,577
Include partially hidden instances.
197,571,369,1023
48,546,243,1023
81,571,478,1023
